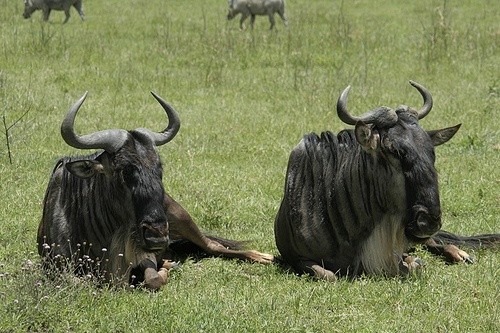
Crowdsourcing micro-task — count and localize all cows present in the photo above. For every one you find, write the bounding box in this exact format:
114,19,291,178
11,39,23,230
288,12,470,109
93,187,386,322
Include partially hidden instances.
22,0,86,25
36,90,274,292
226,0,289,30
274,80,499,285
